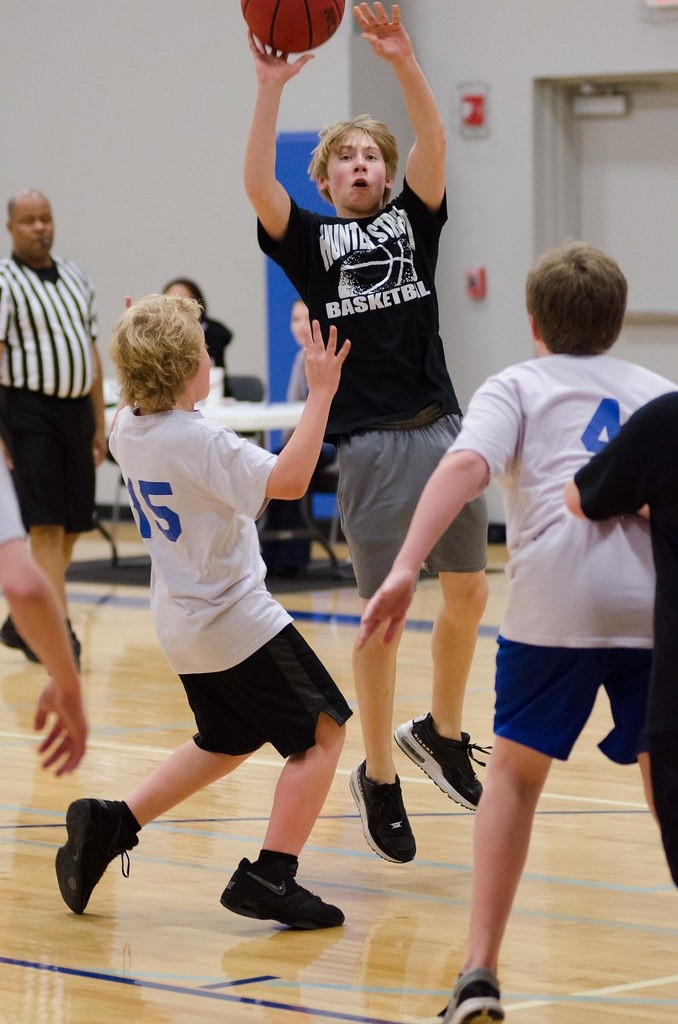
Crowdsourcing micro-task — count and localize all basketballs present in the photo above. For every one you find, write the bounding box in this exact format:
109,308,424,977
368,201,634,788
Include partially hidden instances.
240,0,345,54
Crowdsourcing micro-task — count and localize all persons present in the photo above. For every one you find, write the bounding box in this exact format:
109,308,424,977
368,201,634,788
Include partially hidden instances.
55,294,350,930
353,239,678,1024
243,1,491,871
0,190,109,674
222,913,463,1023
164,277,234,396
271,298,340,473
0,444,85,777
564,390,678,890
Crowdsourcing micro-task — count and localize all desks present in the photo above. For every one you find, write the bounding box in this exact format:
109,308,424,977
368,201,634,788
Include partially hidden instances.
105,402,337,579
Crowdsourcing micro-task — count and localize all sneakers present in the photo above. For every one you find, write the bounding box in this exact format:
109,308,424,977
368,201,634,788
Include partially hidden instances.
437,968,504,1024
220,858,344,928
54,798,141,915
350,759,416,864
394,713,493,811
0,607,81,663
